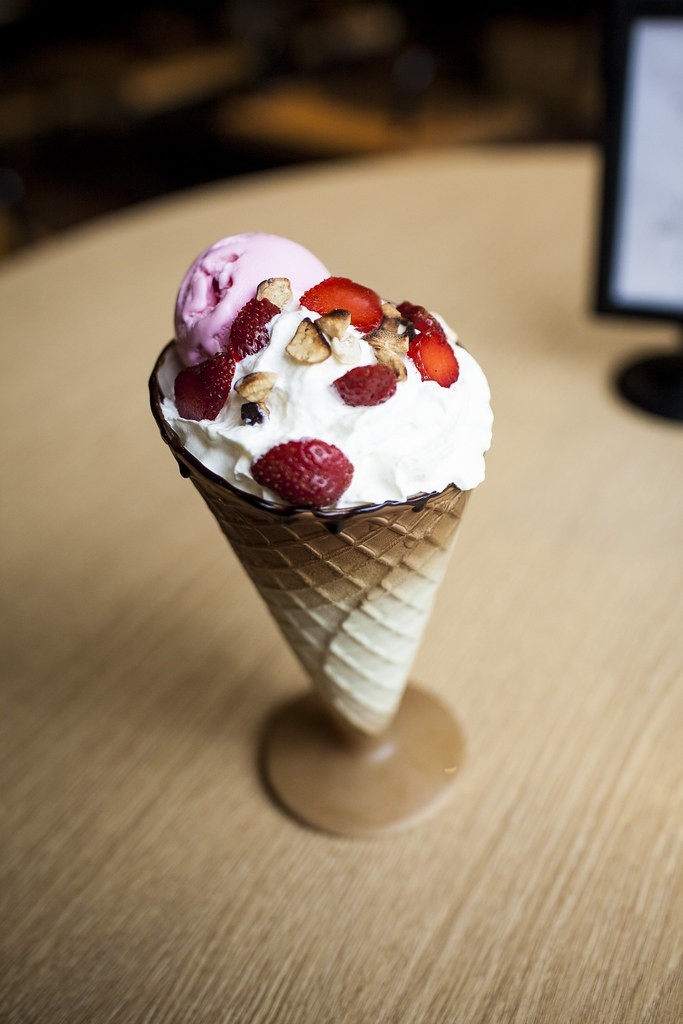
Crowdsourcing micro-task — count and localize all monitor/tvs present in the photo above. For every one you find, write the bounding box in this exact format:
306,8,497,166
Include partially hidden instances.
592,15,683,420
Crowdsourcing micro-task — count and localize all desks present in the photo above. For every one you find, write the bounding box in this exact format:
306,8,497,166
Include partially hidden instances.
0,143,683,1024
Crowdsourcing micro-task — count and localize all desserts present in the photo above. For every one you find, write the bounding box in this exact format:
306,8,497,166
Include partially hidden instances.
156,234,494,739
